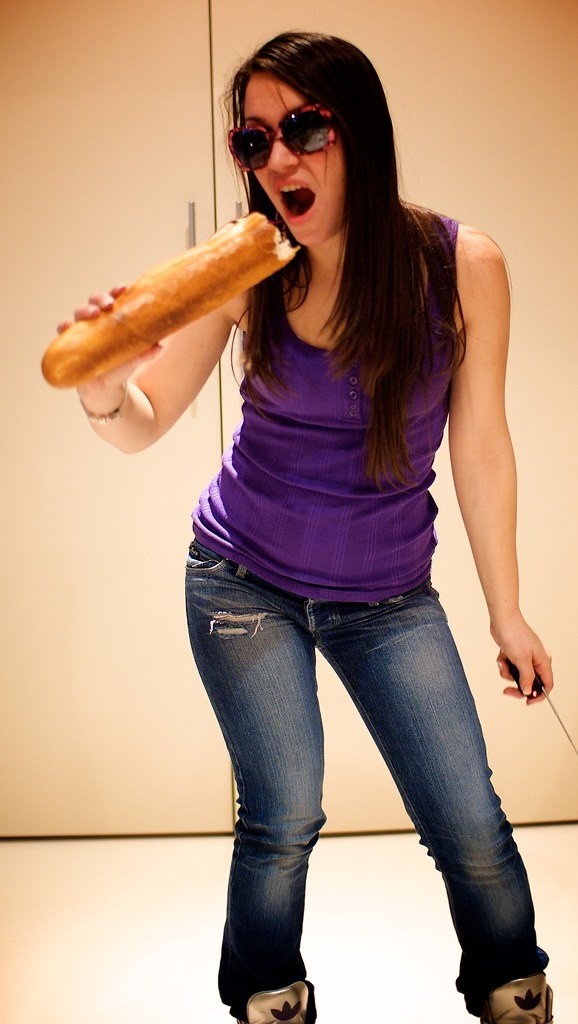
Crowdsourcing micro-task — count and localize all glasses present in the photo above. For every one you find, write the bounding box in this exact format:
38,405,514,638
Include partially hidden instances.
226,102,338,173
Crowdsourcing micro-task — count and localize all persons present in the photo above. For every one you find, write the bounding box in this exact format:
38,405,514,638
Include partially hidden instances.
53,28,562,1023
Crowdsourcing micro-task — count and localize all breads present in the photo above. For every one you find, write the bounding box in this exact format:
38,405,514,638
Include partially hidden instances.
39,210,302,391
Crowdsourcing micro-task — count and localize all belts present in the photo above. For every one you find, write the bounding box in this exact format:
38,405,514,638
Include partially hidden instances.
187,544,434,610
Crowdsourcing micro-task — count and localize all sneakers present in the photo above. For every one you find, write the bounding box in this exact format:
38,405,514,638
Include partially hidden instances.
226,979,319,1024
478,973,555,1024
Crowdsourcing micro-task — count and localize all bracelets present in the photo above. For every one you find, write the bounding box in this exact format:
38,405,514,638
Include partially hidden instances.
79,377,128,425
